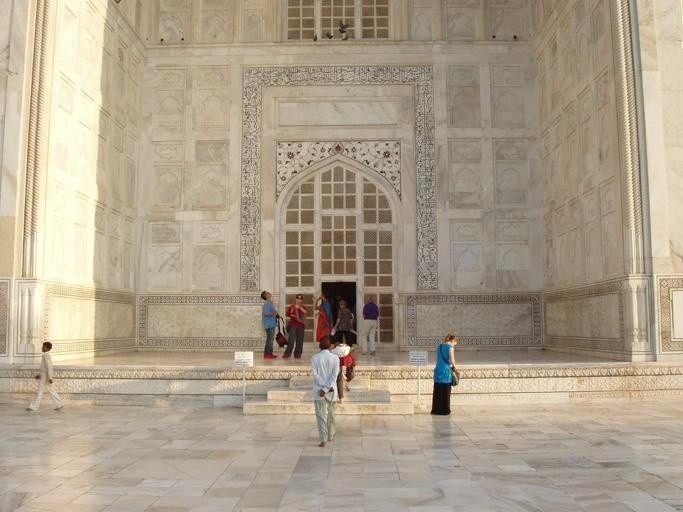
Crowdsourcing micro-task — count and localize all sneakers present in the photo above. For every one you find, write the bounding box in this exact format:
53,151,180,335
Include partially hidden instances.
264,353,302,360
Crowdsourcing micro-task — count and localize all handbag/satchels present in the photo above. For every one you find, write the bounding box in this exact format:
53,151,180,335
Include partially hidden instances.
450,366,460,387
275,316,288,348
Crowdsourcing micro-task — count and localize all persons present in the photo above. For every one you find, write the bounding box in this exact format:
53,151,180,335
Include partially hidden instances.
26,342,64,411
311,337,339,446
261,291,278,358
361,297,378,355
431,335,460,415
283,294,309,358
315,296,353,346
330,342,355,404
